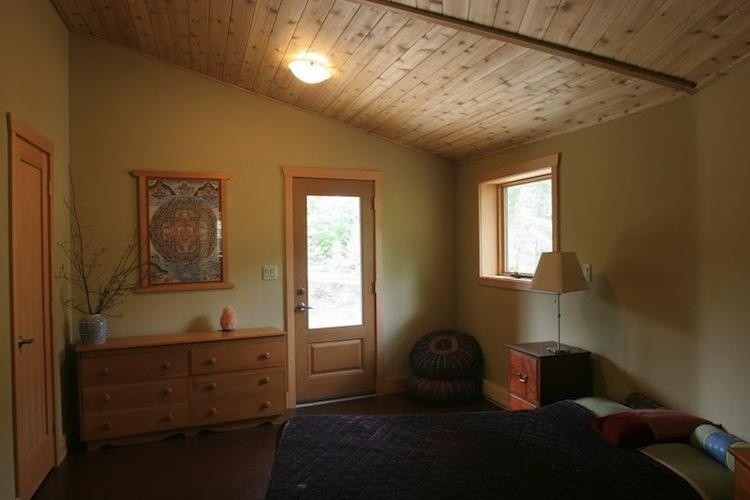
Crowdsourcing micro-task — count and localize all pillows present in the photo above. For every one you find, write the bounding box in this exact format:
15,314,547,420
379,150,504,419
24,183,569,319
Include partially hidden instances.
584,382,749,476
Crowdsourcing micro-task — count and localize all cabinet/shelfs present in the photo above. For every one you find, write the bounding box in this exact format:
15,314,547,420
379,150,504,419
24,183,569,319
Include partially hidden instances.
74,325,288,454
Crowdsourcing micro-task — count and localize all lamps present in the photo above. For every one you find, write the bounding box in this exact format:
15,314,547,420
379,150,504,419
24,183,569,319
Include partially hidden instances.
528,252,591,353
287,57,333,86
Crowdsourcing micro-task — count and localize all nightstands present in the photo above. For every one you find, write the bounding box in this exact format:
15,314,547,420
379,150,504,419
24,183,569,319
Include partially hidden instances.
505,340,592,410
727,448,749,499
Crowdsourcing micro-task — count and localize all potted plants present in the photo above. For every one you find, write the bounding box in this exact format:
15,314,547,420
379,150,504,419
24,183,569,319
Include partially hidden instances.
55,167,150,346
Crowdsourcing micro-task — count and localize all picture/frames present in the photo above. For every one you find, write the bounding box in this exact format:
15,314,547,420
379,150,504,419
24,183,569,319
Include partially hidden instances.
129,169,235,294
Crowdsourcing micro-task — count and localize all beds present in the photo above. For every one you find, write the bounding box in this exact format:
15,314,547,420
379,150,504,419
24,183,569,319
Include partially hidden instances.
265,397,735,499
